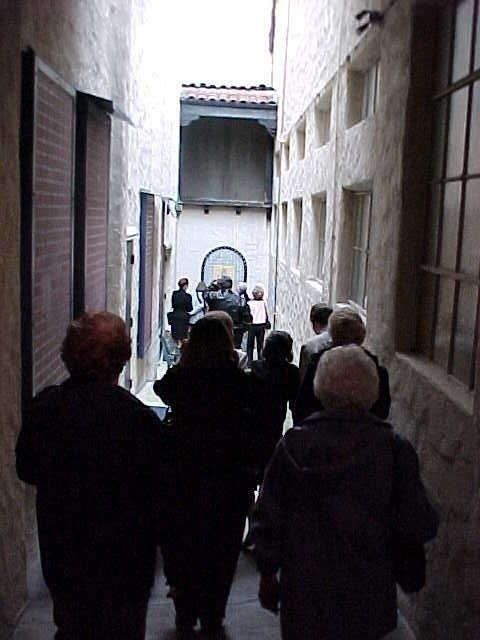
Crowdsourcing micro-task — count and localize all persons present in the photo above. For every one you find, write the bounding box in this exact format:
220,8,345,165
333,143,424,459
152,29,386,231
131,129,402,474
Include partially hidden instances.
16,310,165,640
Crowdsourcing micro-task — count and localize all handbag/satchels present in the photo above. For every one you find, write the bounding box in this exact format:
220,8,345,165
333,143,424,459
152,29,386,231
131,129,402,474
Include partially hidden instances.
394,531,427,594
266,321,271,328
167,312,173,324
